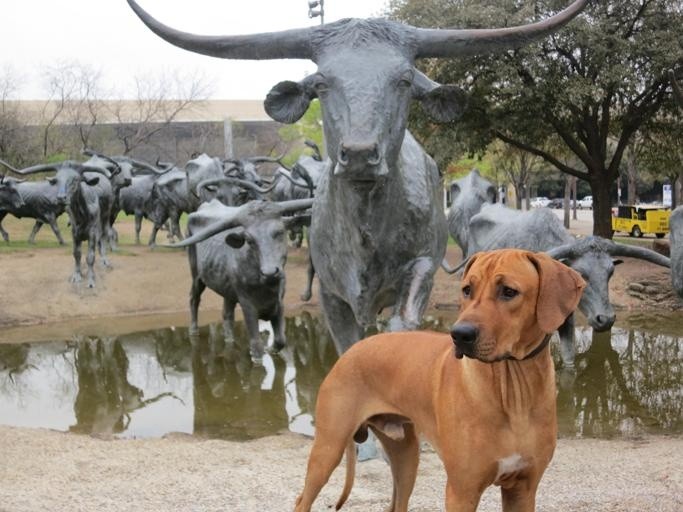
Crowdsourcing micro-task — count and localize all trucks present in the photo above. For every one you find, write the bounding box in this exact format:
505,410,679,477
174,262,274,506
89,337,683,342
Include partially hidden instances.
611,203,672,238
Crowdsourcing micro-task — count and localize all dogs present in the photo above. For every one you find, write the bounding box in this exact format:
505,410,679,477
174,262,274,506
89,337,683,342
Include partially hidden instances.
294,249,588,512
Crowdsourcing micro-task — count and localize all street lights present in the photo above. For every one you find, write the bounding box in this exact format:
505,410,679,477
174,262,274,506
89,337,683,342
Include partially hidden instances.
308,0,324,25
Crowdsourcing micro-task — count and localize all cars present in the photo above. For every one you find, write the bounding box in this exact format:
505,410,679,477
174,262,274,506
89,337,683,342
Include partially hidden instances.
530,195,593,210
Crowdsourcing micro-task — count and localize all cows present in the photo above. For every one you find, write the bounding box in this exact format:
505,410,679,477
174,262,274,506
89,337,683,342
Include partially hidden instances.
442,170,501,262
160,194,328,358
2,155,124,290
1,142,326,246
127,0,589,462
441,204,671,336
